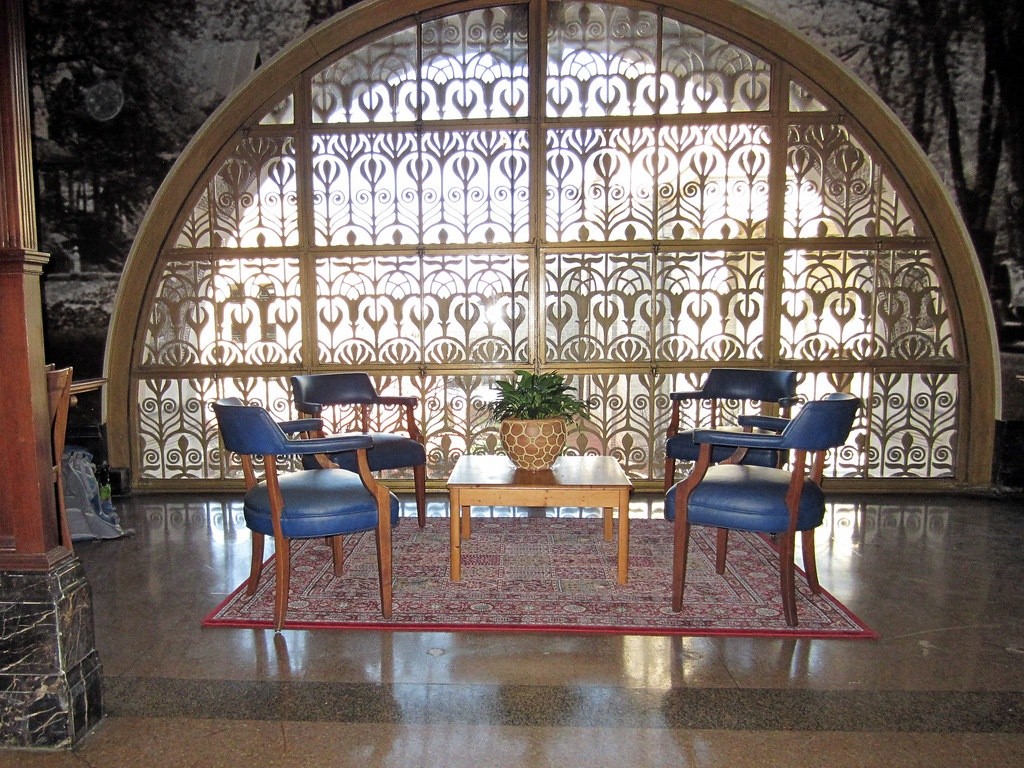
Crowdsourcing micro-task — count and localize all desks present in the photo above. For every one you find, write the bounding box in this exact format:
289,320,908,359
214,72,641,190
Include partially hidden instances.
446,454,634,587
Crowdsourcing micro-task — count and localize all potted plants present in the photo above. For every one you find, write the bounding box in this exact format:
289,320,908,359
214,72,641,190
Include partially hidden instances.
477,370,592,473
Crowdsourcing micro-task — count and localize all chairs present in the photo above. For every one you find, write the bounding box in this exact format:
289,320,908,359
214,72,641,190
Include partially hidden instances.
664,368,798,499
288,372,428,546
44,364,73,559
663,392,862,629
213,397,400,634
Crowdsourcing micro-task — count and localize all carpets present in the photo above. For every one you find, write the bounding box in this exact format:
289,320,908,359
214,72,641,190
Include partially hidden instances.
204,513,881,641
